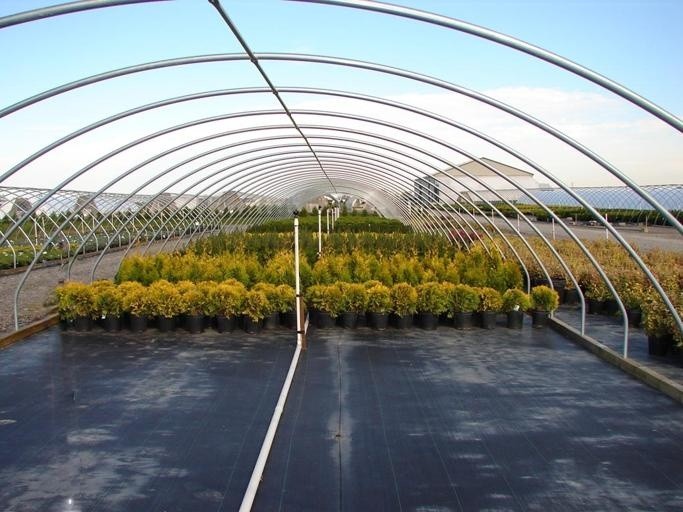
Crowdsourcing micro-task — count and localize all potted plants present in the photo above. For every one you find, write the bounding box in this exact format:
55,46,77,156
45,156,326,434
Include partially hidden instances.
57,279,559,334
555,269,682,365
